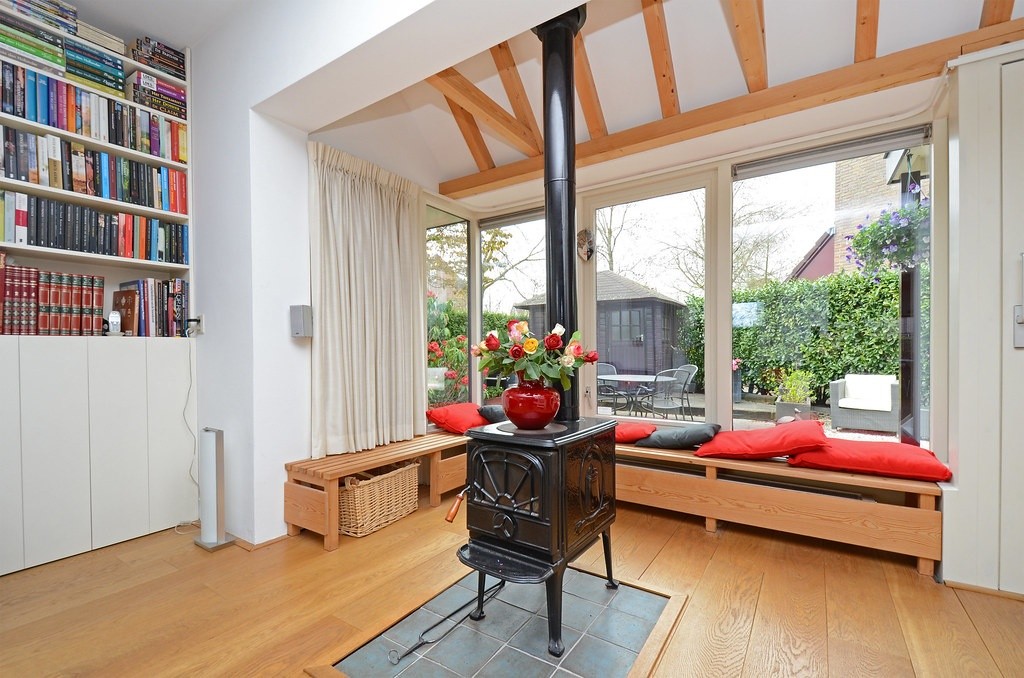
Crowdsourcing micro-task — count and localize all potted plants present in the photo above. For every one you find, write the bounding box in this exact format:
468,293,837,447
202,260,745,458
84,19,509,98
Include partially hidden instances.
774,367,815,422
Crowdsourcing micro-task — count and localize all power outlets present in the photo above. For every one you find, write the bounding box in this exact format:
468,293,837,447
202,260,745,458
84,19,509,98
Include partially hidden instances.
197,314,204,334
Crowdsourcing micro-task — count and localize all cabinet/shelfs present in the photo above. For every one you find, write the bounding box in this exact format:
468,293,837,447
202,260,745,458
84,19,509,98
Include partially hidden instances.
0,4,199,578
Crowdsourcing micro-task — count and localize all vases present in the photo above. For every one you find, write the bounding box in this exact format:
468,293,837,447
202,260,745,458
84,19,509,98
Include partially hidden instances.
501,369,561,430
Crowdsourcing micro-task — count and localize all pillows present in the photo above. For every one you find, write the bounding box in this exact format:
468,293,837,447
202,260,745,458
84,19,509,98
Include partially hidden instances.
693,420,832,460
787,437,952,482
635,423,721,449
426,402,490,435
477,405,509,424
614,421,656,443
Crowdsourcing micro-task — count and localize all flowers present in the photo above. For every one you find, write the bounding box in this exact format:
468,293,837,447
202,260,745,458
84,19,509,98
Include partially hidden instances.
470,319,600,391
733,357,742,370
427,287,490,405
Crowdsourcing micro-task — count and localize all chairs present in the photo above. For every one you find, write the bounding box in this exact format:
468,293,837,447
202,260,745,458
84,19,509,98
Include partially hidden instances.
597,363,628,417
663,364,698,421
635,368,691,420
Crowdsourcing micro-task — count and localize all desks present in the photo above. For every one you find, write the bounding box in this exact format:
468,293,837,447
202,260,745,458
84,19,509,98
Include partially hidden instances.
597,375,677,420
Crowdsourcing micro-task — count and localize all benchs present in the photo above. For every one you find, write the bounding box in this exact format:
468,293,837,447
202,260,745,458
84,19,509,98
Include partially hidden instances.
283,419,940,576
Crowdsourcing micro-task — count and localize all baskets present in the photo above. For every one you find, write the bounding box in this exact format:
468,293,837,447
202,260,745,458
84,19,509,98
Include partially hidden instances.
338,462,420,538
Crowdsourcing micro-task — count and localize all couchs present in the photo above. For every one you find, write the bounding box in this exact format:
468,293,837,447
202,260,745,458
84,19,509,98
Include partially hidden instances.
829,374,900,434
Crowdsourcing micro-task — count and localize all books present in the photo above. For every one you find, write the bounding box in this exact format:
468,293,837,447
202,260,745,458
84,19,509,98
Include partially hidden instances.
0,0,189,337
